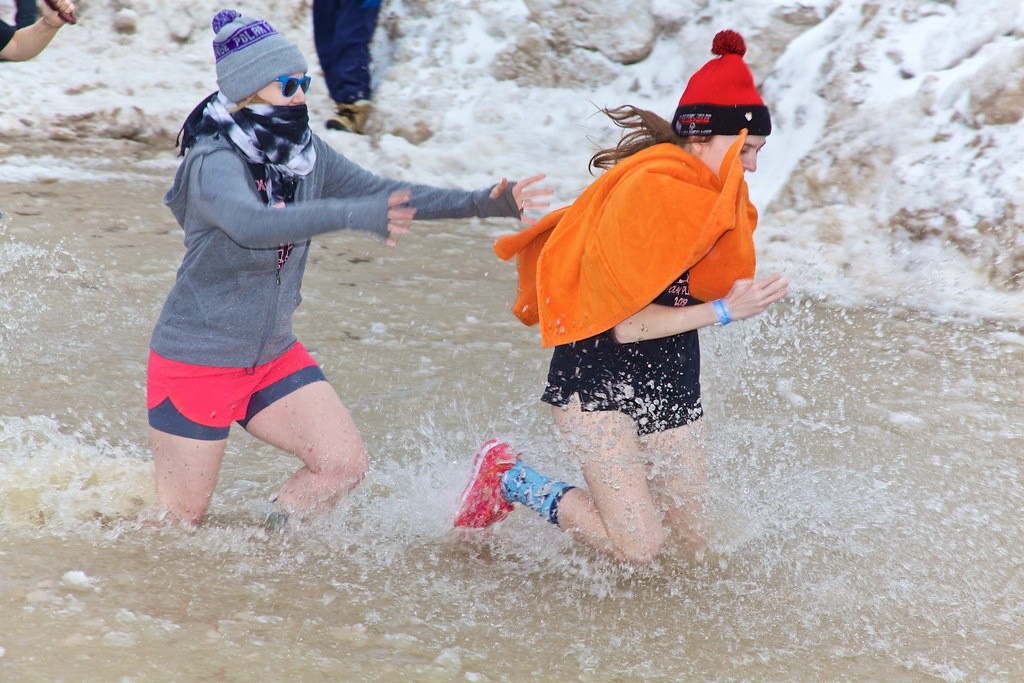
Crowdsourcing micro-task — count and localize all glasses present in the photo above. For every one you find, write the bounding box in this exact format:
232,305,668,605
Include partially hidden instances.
275,75,311,97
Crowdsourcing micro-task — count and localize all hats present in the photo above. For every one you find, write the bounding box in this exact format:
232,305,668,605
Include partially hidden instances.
669,28,770,137
212,10,308,100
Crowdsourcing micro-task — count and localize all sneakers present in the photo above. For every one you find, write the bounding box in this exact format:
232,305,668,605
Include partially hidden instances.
326,102,373,133
458,440,521,535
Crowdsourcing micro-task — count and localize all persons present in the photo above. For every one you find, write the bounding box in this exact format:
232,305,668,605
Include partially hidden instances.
455,29,790,562
0,0,76,62
146,8,553,522
312,0,386,134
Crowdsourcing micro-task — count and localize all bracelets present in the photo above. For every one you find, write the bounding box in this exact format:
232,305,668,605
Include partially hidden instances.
712,299,734,327
